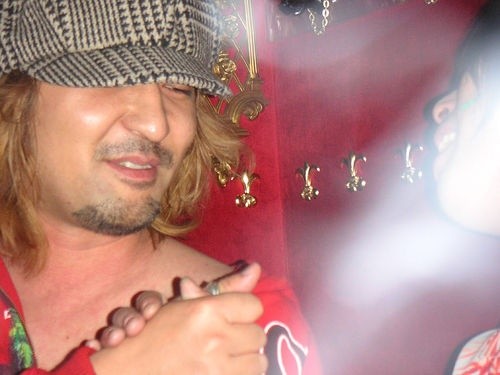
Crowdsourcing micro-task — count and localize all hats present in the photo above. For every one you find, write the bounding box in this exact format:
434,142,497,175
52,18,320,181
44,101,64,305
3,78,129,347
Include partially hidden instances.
0,0,234,102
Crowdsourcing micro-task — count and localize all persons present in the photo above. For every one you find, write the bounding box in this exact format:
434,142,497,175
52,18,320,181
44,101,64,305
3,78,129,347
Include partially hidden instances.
1,0,323,375
77,0,500,374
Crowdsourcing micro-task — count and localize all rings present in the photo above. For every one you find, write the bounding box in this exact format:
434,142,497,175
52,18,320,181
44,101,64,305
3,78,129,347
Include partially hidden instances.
206,278,221,296
